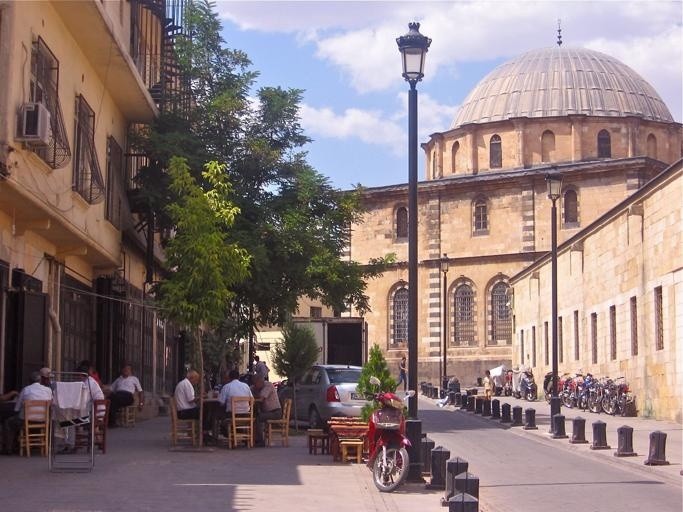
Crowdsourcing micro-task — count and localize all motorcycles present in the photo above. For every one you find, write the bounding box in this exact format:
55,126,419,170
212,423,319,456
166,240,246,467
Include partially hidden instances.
489,365,536,402
543,371,633,415
366,374,416,492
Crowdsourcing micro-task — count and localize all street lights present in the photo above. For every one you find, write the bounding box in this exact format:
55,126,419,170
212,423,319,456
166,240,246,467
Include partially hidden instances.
395,21,432,486
543,163,565,433
439,252,452,399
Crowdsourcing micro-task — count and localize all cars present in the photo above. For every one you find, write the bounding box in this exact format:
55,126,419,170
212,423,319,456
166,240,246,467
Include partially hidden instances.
272,378,287,389
277,364,385,429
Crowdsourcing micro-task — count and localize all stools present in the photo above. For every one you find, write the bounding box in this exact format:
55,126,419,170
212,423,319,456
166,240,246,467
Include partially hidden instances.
307,428,323,448
309,434,329,455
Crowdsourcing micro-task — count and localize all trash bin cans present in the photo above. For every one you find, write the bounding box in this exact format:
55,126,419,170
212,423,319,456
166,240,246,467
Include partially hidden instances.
448,378,460,394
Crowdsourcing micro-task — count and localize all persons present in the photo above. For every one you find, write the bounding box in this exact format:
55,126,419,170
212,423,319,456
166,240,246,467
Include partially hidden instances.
396,357,408,393
102,364,144,429
0,360,105,454
174,370,217,445
482,370,494,401
217,356,282,447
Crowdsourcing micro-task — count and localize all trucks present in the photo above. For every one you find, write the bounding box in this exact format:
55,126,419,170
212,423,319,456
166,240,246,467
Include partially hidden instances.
288,316,367,380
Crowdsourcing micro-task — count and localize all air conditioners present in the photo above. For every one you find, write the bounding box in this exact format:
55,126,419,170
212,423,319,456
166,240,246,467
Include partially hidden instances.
23,103,52,147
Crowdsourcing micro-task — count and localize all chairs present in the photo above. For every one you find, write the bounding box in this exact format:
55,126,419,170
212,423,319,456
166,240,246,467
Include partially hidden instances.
20,372,292,475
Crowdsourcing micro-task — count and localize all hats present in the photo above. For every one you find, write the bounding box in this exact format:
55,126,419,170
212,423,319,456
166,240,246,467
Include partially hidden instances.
40,368,54,377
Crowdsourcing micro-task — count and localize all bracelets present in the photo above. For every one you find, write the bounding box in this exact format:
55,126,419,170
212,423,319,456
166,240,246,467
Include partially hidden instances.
139,402,144,406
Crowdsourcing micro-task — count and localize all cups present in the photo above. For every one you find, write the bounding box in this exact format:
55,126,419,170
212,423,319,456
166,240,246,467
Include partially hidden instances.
208,390,218,399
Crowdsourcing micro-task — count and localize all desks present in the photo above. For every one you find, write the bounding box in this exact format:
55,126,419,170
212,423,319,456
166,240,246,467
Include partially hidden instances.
327,416,369,464
